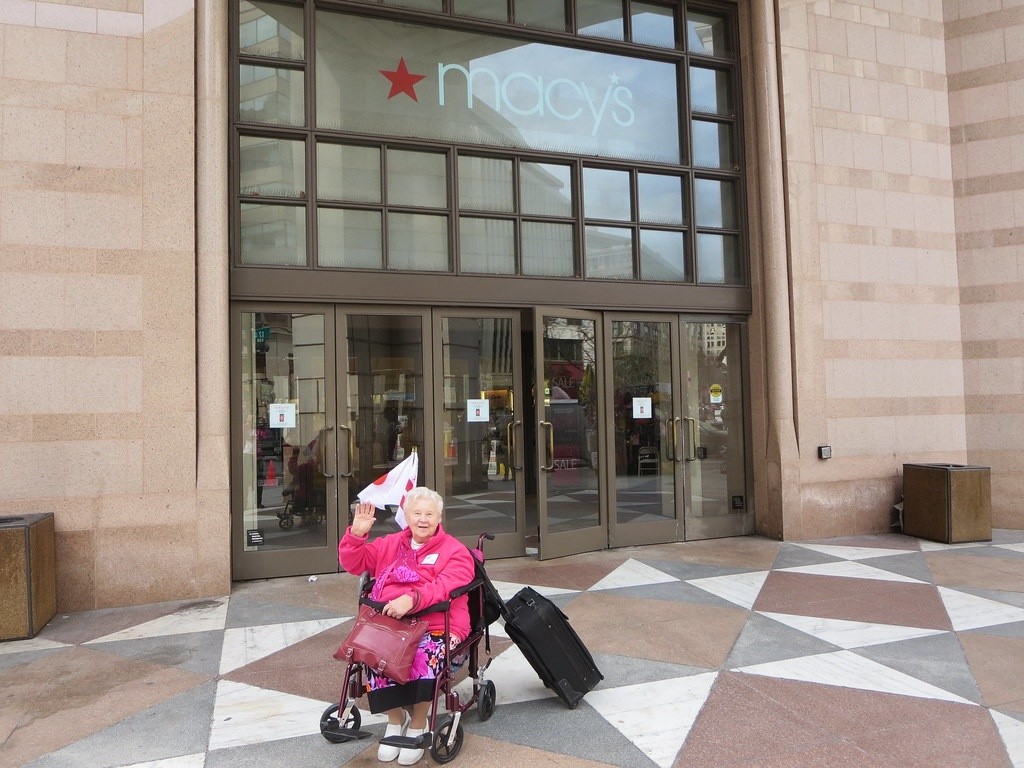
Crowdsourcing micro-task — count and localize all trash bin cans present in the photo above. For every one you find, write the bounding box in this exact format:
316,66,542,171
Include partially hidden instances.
1,513,57,643
903,463,992,543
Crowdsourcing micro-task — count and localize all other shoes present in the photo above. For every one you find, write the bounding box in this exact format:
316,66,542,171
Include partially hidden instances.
389,457,396,461
258,505,266,508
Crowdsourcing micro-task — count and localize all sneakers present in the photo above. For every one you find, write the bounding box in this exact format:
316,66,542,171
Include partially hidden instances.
398,717,429,765
377,710,410,762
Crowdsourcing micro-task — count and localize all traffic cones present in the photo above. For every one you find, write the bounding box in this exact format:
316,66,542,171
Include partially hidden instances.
448,439,457,457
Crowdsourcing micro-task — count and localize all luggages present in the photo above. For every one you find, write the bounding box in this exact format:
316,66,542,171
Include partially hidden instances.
467,549,604,710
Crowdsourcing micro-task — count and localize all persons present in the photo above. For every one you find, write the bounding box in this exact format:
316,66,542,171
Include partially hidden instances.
281,445,325,519
385,416,401,461
494,406,516,481
337,486,475,765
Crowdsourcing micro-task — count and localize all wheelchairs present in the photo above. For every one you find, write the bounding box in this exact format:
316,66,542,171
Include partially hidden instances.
276,478,327,531
318,531,496,765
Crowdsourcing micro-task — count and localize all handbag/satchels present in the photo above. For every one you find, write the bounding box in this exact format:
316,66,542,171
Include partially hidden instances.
333,589,429,685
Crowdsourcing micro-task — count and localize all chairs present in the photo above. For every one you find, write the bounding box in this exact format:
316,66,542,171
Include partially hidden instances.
637,446,662,477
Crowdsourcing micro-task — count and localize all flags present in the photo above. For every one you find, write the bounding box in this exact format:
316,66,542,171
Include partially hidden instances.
356,451,419,530
